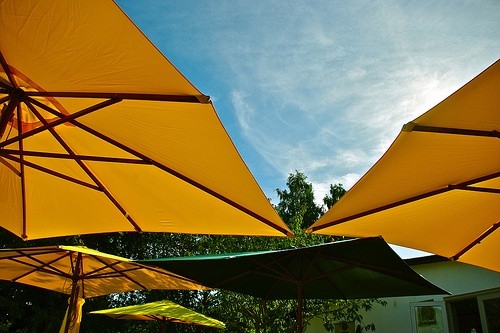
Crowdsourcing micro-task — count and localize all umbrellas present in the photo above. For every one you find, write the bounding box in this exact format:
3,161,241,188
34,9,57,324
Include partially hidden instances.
0,1,293,244
0,244,227,333
304,59,500,275
128,234,454,333
